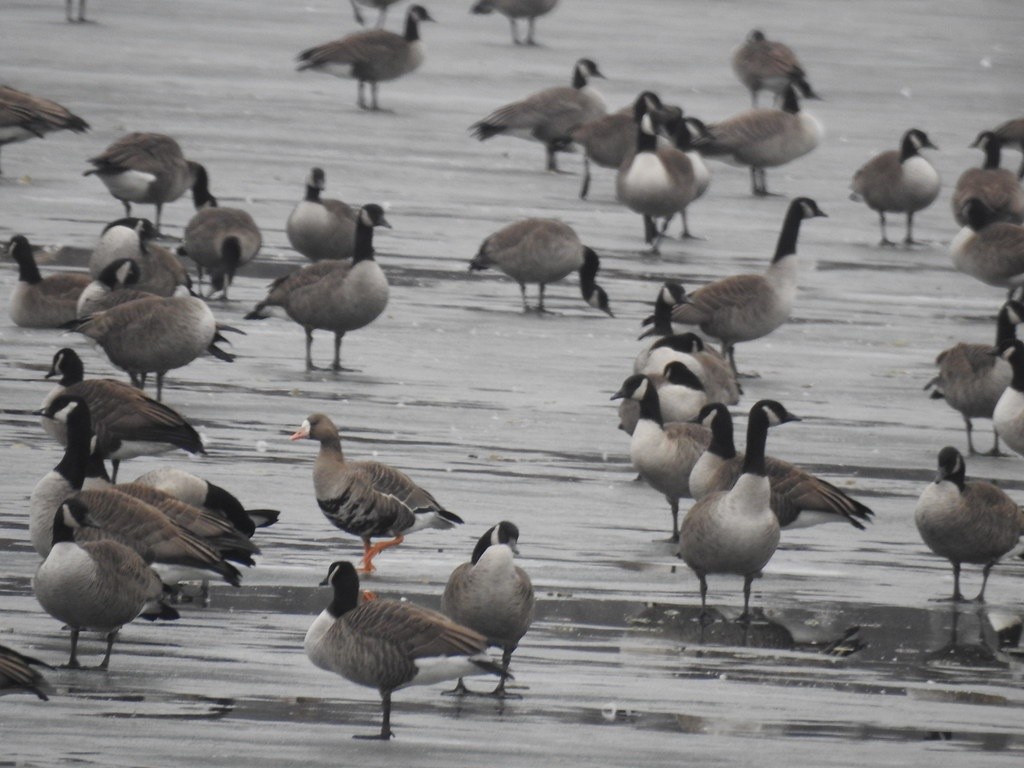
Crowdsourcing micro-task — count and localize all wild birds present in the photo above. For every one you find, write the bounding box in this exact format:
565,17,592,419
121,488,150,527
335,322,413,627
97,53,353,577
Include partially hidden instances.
1,1,1023,747
